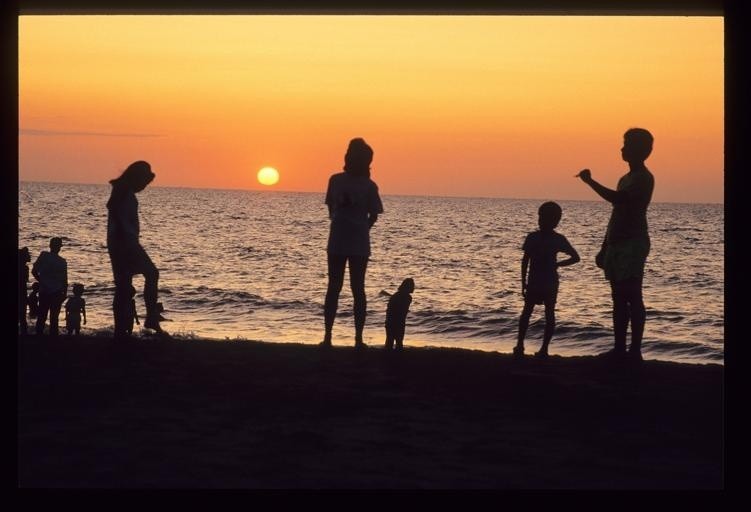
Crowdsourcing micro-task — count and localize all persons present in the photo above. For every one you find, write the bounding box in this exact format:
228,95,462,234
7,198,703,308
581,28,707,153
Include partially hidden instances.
33,237,68,341
18,246,31,333
106,160,165,345
28,281,41,324
379,277,415,351
580,127,655,360
131,285,141,335
513,201,581,356
65,282,86,340
320,137,385,347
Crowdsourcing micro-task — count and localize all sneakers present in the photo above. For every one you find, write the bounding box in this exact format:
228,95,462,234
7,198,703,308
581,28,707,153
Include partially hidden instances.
356,341,368,348
513,341,524,355
535,344,548,357
320,337,331,345
598,347,644,359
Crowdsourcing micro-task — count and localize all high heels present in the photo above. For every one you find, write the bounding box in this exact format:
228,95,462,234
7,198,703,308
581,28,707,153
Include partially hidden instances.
142,319,168,334
